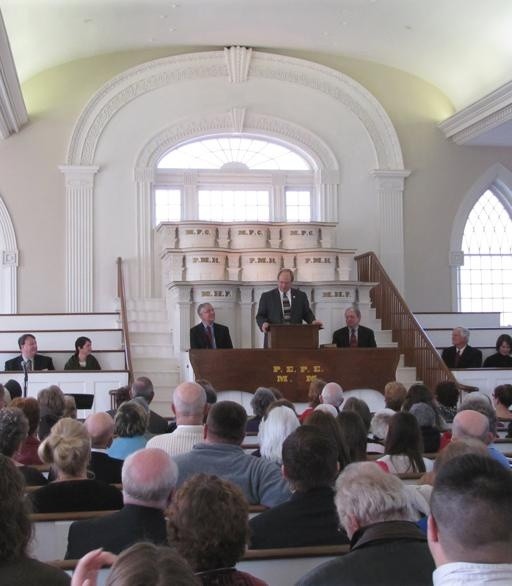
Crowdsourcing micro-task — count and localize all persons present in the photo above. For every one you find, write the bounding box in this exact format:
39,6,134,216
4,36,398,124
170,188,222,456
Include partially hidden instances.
247,425,351,549
169,380,216,432
190,303,233,348
63,337,101,370
5,334,55,372
427,453,512,586
371,409,396,443
65,448,180,568
440,391,499,451
256,268,323,348
402,384,449,431
319,382,344,413
452,411,511,469
332,307,377,347
494,384,512,428
337,410,369,462
291,461,435,586
296,380,326,424
436,381,460,413
166,474,270,586
107,386,129,418
84,412,124,485
25,416,124,513
384,381,409,414
7,396,45,465
376,414,433,473
418,436,491,485
130,397,155,440
0,406,50,485
246,388,276,432
107,400,149,460
484,334,512,369
38,385,66,440
71,544,202,586
250,406,300,465
144,381,207,456
0,498,71,586
130,376,168,435
342,397,385,454
441,327,482,368
410,403,453,452
302,411,351,470
172,400,291,508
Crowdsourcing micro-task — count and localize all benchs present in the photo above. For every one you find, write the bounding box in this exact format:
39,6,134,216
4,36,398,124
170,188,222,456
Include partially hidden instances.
26,506,268,563
413,311,501,329
0,350,127,370
0,313,121,330
45,545,351,585
422,327,510,347
1,330,123,349
439,348,495,367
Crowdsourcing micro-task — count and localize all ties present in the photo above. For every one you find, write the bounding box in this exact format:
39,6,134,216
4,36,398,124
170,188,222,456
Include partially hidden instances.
283,292,291,322
207,325,212,344
454,351,460,367
27,360,32,371
350,329,357,347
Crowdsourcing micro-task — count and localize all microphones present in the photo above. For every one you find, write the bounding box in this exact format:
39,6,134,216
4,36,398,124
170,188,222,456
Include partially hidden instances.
21,361,28,376
291,290,298,324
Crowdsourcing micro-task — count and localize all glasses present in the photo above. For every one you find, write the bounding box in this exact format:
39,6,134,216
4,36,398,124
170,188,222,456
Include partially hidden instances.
492,393,495,397
